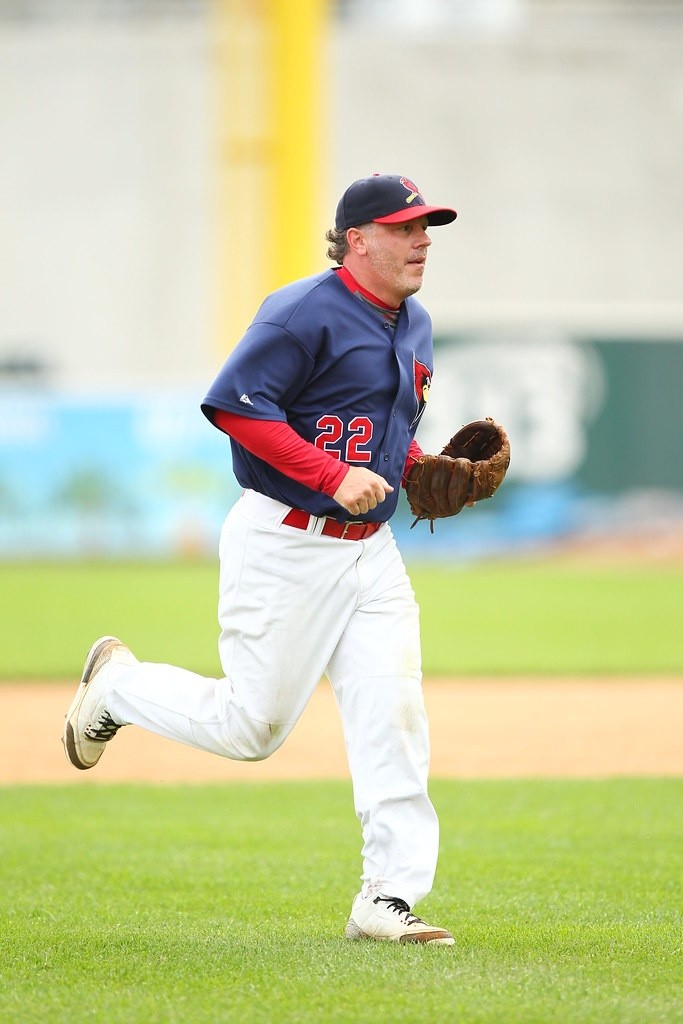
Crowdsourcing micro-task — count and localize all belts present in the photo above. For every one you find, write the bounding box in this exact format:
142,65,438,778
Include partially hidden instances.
281,508,382,541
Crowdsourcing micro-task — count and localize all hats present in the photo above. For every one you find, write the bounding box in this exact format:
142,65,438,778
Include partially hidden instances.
336,173,457,233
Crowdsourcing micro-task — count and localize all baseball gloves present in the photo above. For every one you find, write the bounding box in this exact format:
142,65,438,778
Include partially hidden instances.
408,416,510,538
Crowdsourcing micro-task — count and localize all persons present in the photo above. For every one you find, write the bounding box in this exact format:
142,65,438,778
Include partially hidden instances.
64,172,471,945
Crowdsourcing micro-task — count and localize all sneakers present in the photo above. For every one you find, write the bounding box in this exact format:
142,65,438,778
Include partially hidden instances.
62,636,132,770
345,892,455,947
